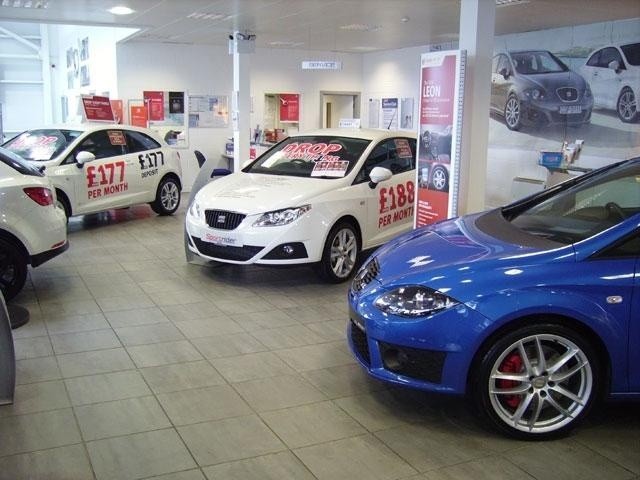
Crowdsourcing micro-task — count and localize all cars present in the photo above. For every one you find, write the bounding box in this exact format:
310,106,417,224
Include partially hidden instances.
579,40,640,124
184,110,420,286
489,49,593,131
0,123,185,215
347,154,640,440
0,147,69,304
418,153,450,192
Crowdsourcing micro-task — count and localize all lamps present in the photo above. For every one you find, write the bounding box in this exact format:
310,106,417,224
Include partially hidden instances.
236,32,256,54
228,32,234,54
